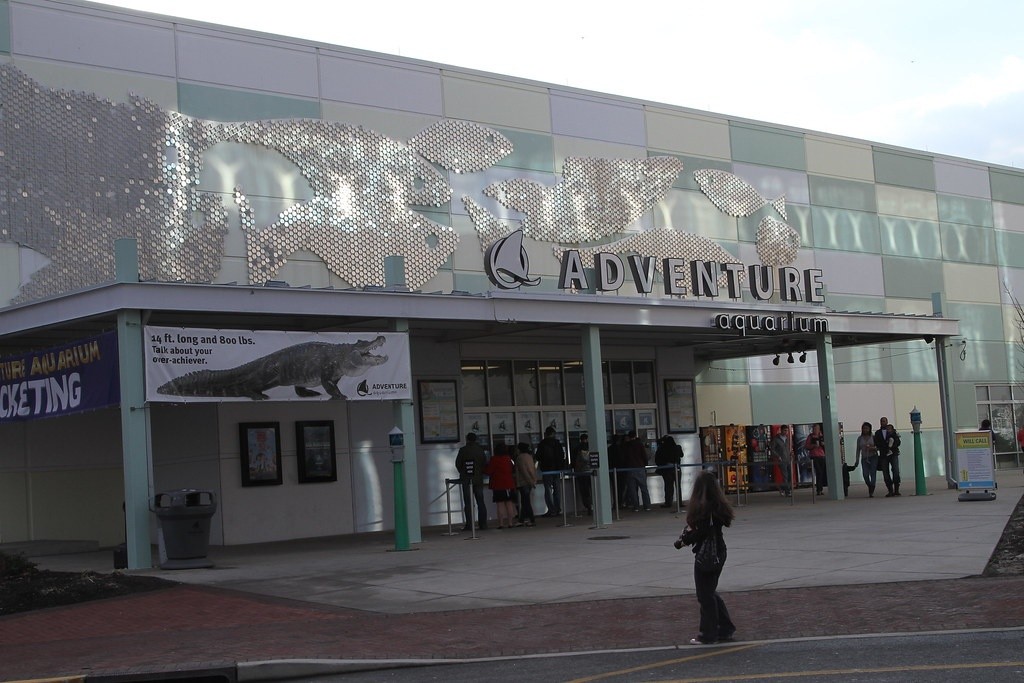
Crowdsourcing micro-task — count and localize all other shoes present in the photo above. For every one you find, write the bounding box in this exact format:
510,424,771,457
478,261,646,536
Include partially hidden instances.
690,630,736,644
785,489,792,497
885,492,901,497
779,487,784,496
887,450,893,456
496,526,505,529
680,502,688,507
514,521,536,528
508,525,512,528
475,526,488,530
460,527,472,530
544,512,558,517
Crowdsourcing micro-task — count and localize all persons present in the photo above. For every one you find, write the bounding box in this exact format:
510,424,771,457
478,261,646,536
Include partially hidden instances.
979,420,996,446
456,433,488,531
513,443,536,528
805,424,826,495
1017,425,1024,473
842,416,901,497
768,425,792,497
574,430,652,515
536,427,564,517
674,469,737,644
655,435,687,508
484,443,516,529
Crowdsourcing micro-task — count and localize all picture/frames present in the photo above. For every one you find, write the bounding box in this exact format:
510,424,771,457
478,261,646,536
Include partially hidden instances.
295,420,337,484
238,422,284,488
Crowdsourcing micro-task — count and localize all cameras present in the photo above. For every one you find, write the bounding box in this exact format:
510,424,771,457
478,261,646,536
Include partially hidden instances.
674,539,684,550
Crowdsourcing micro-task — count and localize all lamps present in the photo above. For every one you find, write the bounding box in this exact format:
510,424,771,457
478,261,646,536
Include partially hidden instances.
551,419,557,430
388,426,405,462
574,418,580,429
788,352,794,363
472,421,480,432
923,335,934,344
498,420,506,432
909,406,922,433
524,420,531,431
799,351,807,363
773,353,780,365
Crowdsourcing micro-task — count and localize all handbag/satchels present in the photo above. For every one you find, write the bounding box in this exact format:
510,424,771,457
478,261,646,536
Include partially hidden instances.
695,511,723,574
876,450,888,471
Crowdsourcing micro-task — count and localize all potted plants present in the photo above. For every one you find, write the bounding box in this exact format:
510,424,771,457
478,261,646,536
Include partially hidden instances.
0,549,38,616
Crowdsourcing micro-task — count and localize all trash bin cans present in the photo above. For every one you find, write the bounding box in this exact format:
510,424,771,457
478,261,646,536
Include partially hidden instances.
148,489,218,560
